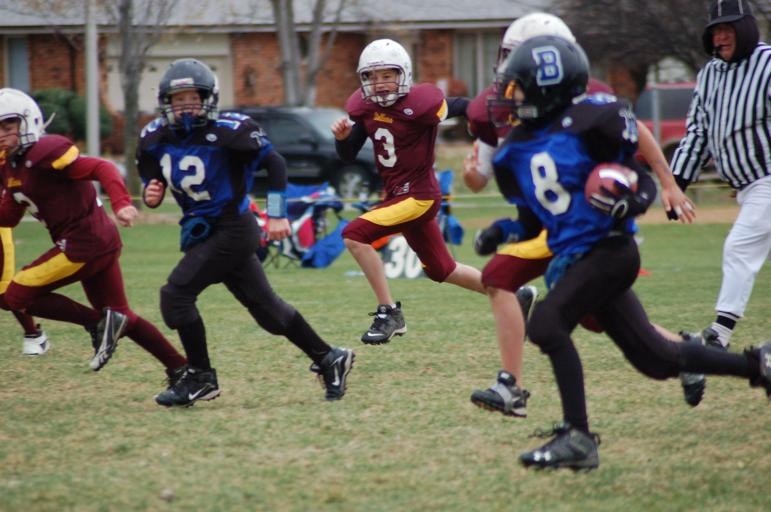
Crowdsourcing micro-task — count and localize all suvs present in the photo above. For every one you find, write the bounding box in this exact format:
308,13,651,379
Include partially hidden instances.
217,104,391,201
630,80,715,169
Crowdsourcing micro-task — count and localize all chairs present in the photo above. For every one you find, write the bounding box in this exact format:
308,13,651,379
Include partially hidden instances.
263,182,332,273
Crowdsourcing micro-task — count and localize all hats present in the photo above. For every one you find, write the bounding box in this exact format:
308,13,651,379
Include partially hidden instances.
704,0,756,28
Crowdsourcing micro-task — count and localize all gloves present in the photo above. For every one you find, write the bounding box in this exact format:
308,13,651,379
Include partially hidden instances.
591,181,645,219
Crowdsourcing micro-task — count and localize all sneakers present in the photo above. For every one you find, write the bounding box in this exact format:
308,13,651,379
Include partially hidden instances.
516,287,538,330
310,346,355,400
519,427,599,470
751,343,770,394
363,302,407,346
21,332,52,355
680,329,731,407
87,310,126,372
472,371,530,417
156,366,220,409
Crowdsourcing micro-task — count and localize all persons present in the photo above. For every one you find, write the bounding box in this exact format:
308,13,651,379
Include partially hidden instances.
0,87,189,392
473,34,771,473
0,226,51,357
463,11,709,417
135,57,355,410
330,38,538,345
664,0,771,352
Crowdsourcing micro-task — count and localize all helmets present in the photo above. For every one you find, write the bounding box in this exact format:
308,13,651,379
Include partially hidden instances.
486,12,588,129
0,86,43,165
357,39,411,108
158,60,219,132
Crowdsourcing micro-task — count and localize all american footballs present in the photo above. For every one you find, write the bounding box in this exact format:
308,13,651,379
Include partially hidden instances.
585,163,640,206
